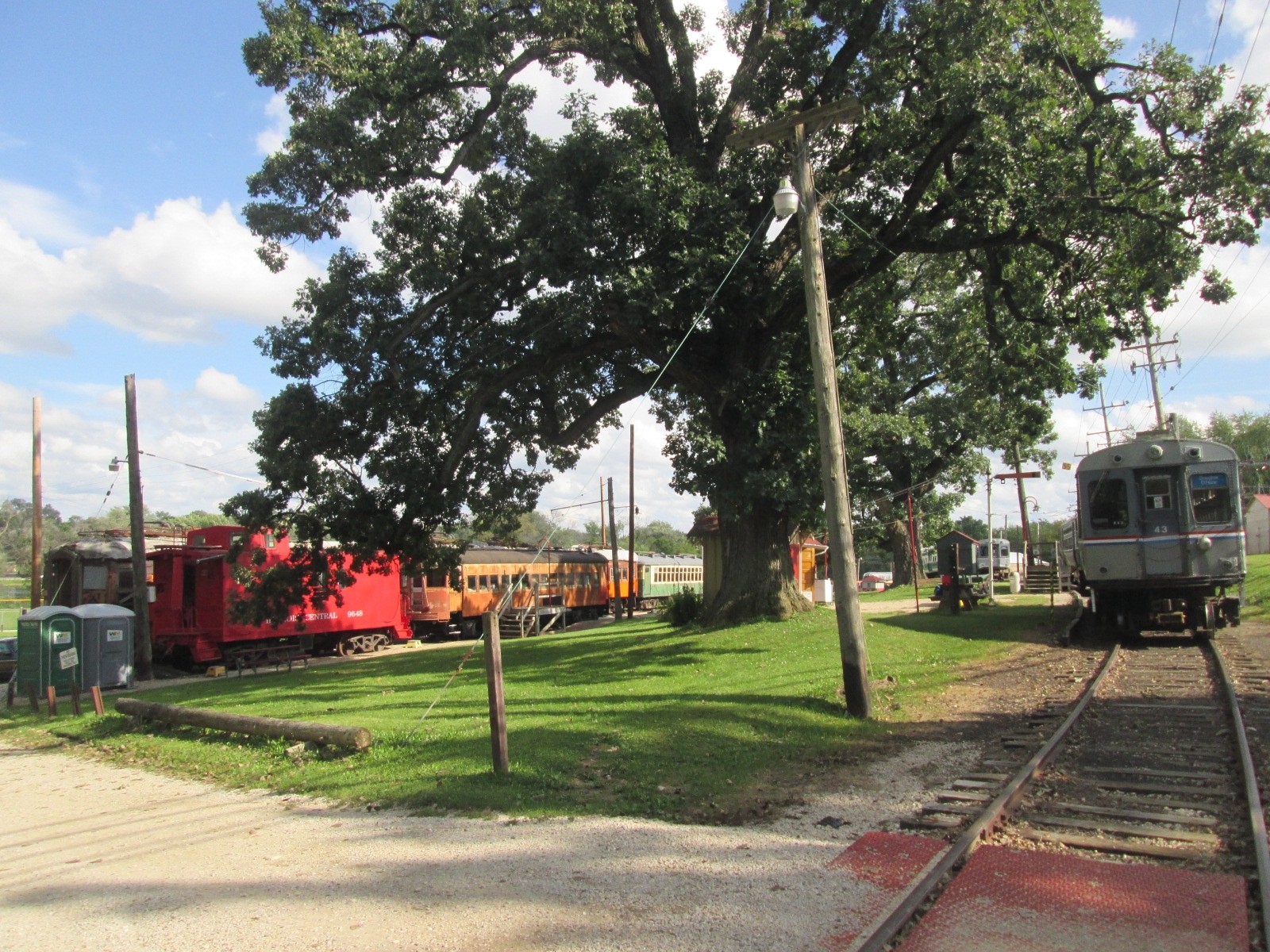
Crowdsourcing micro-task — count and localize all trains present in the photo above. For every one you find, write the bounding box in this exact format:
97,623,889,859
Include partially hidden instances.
1061,432,1246,642
42,520,708,673
